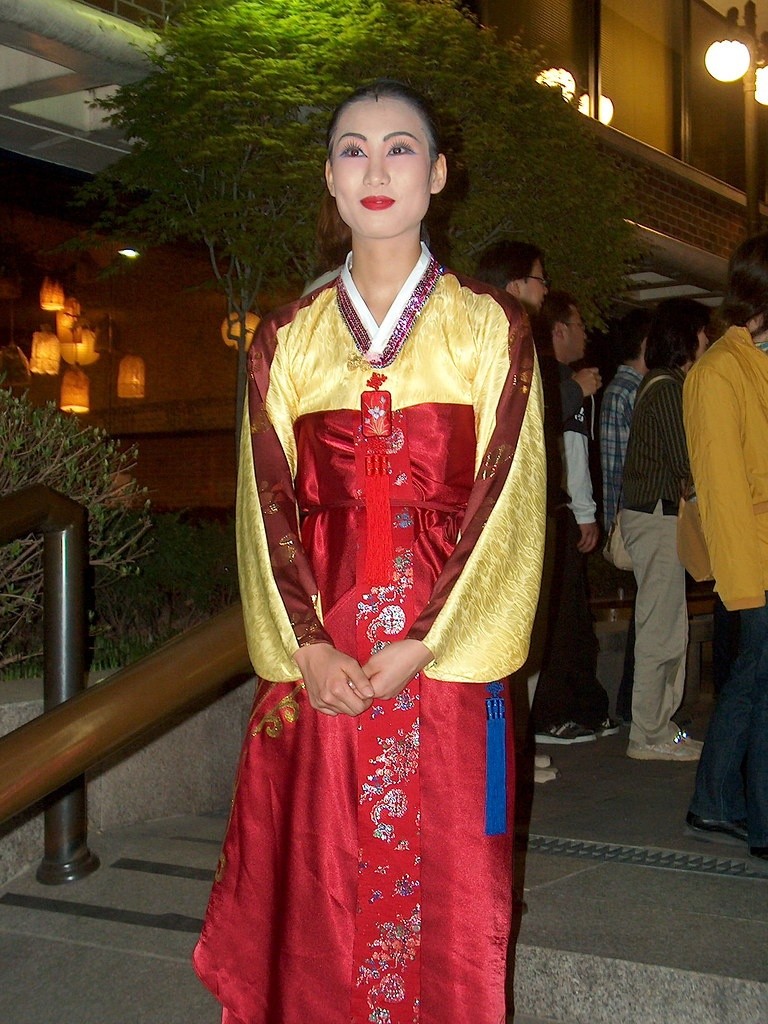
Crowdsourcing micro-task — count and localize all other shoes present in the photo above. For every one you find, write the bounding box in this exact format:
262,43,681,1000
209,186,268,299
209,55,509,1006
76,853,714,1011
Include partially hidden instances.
747,843,768,864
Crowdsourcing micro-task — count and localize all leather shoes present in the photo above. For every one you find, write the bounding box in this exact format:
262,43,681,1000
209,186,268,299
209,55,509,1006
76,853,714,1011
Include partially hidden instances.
686,811,748,842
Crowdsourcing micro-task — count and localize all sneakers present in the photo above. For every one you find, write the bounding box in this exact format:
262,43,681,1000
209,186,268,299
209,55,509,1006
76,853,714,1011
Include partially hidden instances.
575,716,620,737
533,719,597,744
626,738,701,761
674,731,705,749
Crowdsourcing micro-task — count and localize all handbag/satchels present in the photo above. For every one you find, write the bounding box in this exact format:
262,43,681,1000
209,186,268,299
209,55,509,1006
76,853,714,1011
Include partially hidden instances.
603,520,633,573
677,490,714,583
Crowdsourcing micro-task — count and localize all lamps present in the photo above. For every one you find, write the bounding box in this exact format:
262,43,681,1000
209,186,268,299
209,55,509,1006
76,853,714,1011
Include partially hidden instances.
39,274,65,311
55,296,84,344
58,328,101,365
60,367,91,414
0,307,32,387
116,354,147,400
28,323,63,376
220,307,263,353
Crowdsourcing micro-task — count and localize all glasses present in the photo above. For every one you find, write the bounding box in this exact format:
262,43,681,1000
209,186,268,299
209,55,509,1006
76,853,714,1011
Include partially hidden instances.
513,273,550,291
563,320,587,333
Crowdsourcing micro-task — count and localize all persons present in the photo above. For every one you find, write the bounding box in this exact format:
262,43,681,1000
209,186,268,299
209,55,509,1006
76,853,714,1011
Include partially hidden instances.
194,84,546,1024
474,232,768,861
